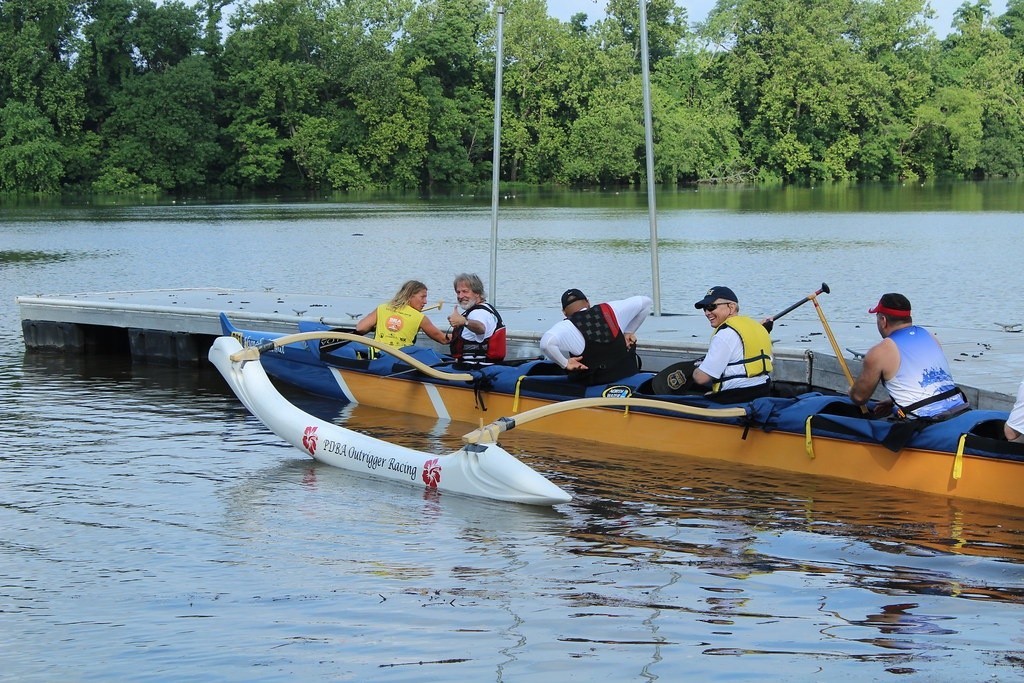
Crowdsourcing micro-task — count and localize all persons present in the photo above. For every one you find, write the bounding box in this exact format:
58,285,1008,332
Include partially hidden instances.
845,292,966,423
693,285,774,401
355,279,452,361
446,272,503,368
539,288,654,386
1004,378,1024,443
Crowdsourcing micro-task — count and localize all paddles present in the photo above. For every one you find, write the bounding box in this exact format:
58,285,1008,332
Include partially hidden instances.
502,355,545,360
807,290,872,416
319,299,445,353
650,281,832,399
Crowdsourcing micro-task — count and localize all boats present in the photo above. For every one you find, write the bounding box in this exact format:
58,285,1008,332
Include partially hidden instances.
205,311,1023,509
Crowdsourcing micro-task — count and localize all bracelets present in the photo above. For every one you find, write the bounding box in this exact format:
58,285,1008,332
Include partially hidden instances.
464,319,469,327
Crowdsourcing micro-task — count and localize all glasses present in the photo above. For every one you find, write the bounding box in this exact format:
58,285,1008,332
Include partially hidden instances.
703,302,731,312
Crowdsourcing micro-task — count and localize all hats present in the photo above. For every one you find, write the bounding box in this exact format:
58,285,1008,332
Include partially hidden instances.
561,288,587,310
868,293,912,317
694,285,739,309
